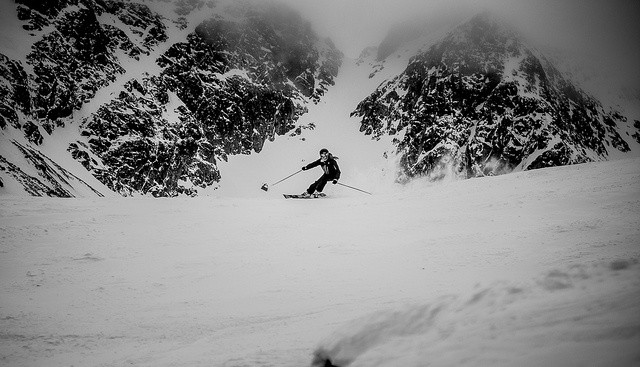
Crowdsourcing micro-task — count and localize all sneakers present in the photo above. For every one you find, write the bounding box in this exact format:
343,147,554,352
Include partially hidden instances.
300,192,311,200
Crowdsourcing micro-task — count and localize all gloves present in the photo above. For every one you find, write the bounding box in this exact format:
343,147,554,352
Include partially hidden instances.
302,167,305,170
333,180,337,184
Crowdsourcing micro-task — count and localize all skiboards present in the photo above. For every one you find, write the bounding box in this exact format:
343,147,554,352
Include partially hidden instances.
282,192,327,199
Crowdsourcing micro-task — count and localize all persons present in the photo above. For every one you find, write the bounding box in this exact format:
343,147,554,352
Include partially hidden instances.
302,148,341,198
